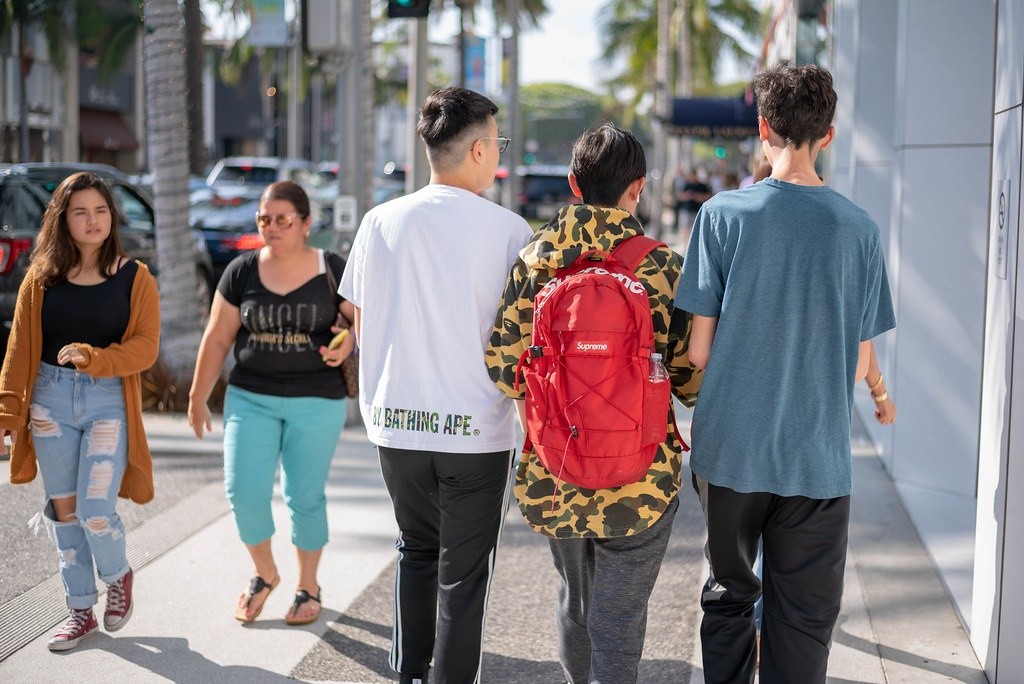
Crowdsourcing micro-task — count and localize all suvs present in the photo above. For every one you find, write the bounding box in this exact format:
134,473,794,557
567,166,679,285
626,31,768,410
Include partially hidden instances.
0,161,216,345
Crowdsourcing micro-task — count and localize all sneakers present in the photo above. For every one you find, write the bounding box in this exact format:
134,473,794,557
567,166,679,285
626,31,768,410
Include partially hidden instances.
103,566,134,632
47,606,100,650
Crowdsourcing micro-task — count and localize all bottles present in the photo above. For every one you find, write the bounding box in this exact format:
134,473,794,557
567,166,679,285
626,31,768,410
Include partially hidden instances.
648,353,669,383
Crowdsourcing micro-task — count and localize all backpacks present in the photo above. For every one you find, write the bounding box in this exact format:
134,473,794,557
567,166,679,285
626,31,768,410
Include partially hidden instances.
515,236,690,490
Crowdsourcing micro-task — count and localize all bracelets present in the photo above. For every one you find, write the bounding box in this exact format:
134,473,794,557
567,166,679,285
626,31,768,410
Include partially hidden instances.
871,388,888,401
870,373,882,391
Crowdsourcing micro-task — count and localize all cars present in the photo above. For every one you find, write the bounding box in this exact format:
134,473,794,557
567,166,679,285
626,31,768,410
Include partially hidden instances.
128,171,209,214
190,157,582,268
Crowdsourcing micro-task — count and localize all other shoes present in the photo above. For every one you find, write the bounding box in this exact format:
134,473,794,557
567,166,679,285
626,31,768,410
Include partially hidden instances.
398,673,428,684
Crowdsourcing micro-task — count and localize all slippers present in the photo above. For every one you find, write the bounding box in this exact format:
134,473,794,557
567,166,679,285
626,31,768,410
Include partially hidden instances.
234,575,280,622
286,586,321,624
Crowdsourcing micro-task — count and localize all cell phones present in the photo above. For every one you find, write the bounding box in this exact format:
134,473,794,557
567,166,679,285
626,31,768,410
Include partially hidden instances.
324,327,349,362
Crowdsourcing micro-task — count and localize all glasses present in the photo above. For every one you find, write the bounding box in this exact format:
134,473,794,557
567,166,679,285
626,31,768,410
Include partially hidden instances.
471,135,510,153
255,210,307,230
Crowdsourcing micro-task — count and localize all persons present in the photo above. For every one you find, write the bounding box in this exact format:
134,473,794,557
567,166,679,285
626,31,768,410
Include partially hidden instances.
0,170,161,650
672,154,896,667
674,58,895,684
338,86,534,684
188,181,357,624
484,125,705,684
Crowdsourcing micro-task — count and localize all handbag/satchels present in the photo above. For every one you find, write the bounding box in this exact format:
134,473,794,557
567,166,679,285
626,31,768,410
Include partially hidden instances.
324,250,360,398
